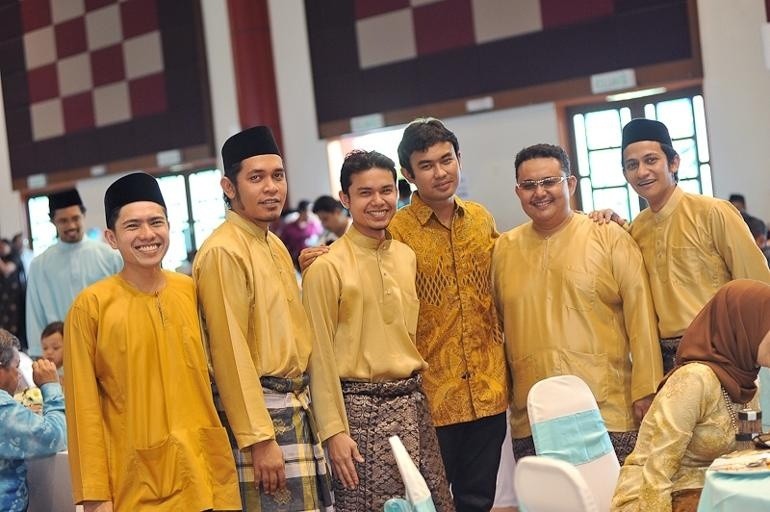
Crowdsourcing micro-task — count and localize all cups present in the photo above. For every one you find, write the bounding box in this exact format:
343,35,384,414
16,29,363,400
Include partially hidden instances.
737,408,762,451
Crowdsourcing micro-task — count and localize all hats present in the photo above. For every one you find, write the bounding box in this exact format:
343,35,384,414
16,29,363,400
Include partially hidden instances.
622,118,672,150
104,172,166,221
49,188,82,210
221,125,282,169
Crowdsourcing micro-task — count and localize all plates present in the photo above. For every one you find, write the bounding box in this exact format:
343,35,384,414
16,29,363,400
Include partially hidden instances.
716,454,770,475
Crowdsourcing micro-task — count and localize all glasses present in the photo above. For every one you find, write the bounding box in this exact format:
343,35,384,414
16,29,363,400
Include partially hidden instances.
515,176,570,190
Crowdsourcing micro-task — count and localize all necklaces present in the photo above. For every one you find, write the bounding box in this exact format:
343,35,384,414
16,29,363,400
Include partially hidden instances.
721,385,749,431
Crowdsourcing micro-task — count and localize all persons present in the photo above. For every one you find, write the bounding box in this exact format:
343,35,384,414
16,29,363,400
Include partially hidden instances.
0,238,26,350
0,327,30,392
0,343,68,512
586,118,770,375
301,149,458,512
728,194,767,249
609,278,770,512
24,183,123,358
312,194,353,247
60,169,245,512
396,178,413,208
12,232,36,288
297,117,501,512
491,143,665,467
190,124,337,512
281,199,322,267
40,320,65,384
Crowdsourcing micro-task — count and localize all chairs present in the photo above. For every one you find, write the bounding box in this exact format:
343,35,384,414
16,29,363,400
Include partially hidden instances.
513,456,595,512
390,435,442,512
527,375,621,512
